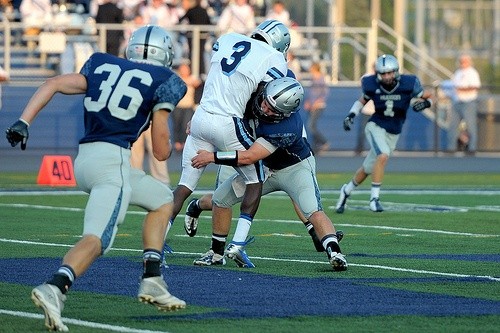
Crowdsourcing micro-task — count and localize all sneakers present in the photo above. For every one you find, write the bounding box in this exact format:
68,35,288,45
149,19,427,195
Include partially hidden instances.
330,252,348,270
336,184,350,213
226,244,256,268
369,198,383,211
193,249,226,266
184,198,202,236
314,231,344,252
160,257,169,269
32,283,69,332
138,274,185,311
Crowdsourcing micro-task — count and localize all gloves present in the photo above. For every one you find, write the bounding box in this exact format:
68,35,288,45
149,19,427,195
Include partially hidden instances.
344,113,355,131
412,101,431,112
5,120,29,150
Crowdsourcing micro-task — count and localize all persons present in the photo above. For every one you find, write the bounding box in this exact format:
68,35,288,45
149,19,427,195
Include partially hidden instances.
184,166,344,251
5,24,187,332
336,54,437,214
0,0,303,83
191,70,348,270
161,19,291,268
302,63,329,155
444,54,481,157
354,62,378,160
130,121,170,186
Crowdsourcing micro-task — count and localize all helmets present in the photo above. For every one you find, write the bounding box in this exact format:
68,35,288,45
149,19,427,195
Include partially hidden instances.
125,25,174,67
263,77,305,114
375,54,399,81
250,19,291,54
